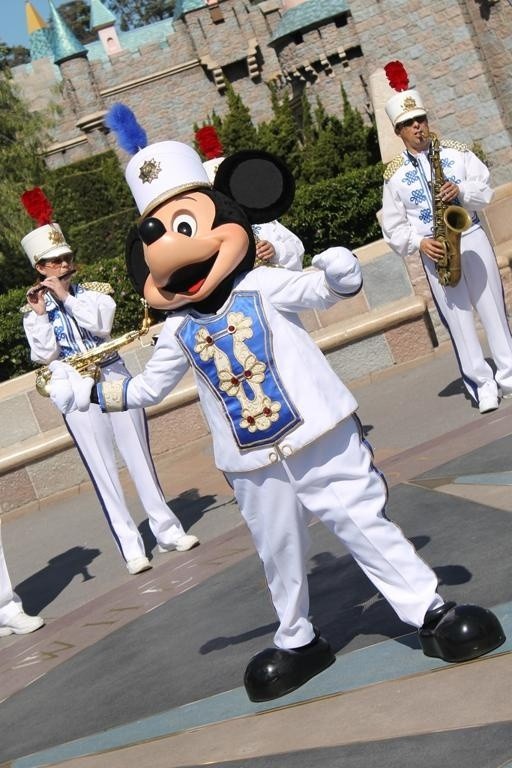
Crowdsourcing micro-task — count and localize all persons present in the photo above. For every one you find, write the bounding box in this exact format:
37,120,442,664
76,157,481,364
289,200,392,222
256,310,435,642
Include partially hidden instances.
375,89,512,414
20,222,199,575
202,155,304,272
0,537,45,639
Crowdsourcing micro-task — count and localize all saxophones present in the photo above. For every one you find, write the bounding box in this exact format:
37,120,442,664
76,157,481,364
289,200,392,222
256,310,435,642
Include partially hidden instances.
35,299,150,398
420,129,472,286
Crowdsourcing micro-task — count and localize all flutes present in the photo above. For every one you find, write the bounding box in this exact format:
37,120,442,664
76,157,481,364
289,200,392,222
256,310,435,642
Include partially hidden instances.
27,269,79,296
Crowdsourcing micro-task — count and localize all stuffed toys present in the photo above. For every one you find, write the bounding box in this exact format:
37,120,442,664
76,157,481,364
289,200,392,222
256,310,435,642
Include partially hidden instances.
41,140,506,701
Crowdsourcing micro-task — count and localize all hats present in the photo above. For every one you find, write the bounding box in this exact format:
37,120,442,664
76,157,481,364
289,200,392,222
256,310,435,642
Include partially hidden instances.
202,157,226,185
385,90,428,130
20,223,74,269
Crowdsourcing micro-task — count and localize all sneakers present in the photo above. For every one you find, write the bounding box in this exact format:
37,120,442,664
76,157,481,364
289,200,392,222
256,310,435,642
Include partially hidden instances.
479,395,499,413
159,535,199,553
0,610,43,636
126,557,152,574
